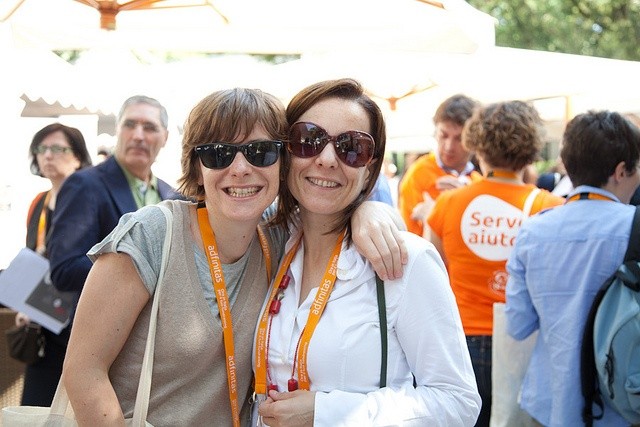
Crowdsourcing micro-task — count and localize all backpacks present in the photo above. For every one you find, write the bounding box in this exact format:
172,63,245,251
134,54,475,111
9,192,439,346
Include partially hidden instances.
581,204,640,427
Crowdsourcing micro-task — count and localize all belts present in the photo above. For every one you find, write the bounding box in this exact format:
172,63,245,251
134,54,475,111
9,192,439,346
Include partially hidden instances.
466,336,492,343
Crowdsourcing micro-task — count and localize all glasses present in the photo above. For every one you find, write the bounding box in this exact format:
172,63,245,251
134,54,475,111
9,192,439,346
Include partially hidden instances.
37,144,73,154
287,122,376,168
194,140,284,169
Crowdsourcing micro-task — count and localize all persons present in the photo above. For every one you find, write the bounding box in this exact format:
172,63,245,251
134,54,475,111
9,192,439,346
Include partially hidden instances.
398,95,640,427
252,79,482,427
0,89,408,427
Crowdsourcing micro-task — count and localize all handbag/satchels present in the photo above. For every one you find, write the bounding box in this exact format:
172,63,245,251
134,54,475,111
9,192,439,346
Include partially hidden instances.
0,406,154,427
5,320,44,364
489,300,553,427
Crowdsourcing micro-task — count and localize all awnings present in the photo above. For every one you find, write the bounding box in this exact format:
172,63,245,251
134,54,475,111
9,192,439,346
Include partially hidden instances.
1,0,640,154
